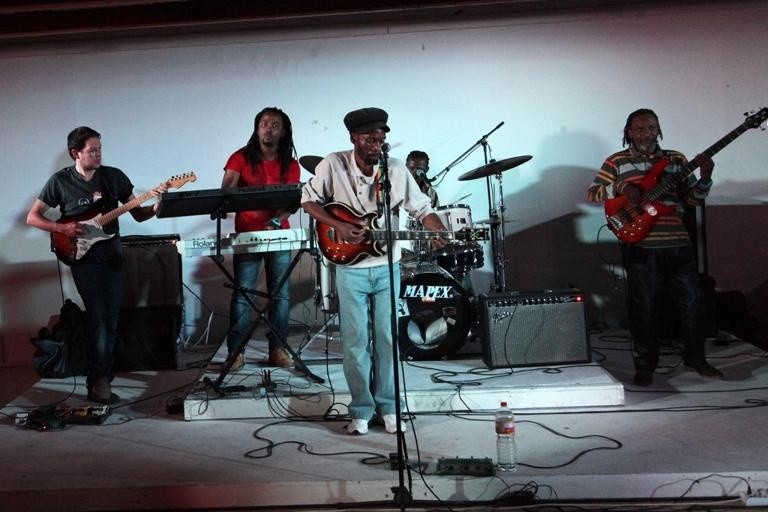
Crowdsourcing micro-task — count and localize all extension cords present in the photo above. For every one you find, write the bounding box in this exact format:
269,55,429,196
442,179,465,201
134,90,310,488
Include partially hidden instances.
257,381,277,392
14,405,113,428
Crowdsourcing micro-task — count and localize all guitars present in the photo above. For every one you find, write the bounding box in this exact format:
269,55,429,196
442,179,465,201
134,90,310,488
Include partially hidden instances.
606,106,768,245
50,171,196,267
315,201,490,266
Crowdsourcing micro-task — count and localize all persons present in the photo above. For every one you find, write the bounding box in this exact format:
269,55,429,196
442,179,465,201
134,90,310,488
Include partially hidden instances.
301,106,451,435
216,106,300,372
404,149,443,210
586,109,722,386
26,127,171,403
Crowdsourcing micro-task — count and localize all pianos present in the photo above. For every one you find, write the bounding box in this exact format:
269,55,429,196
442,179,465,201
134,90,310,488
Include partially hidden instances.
175,227,319,258
156,182,306,218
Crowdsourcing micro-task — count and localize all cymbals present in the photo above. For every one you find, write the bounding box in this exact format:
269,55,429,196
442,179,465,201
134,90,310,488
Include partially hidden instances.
299,156,323,176
476,217,515,224
458,156,533,181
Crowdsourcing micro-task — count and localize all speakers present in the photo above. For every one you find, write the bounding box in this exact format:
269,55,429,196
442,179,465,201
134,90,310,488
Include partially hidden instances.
478,285,592,370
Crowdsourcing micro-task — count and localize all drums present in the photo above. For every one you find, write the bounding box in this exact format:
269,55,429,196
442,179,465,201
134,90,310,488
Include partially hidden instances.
429,245,483,272
398,261,475,361
424,203,475,246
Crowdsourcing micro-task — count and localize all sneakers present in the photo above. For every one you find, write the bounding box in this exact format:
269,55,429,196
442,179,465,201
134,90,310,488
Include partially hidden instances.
347,418,369,434
87,390,120,404
681,354,724,379
221,349,245,371
269,346,292,367
377,413,407,434
634,365,653,386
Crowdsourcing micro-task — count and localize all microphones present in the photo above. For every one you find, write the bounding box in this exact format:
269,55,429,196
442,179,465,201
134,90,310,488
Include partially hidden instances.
380,142,391,152
416,169,432,187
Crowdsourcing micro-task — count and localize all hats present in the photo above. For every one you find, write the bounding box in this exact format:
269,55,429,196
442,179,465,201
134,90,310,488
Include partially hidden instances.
344,108,390,134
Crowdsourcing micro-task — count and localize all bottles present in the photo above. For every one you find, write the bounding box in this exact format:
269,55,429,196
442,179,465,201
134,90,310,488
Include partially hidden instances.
495,400,517,473
